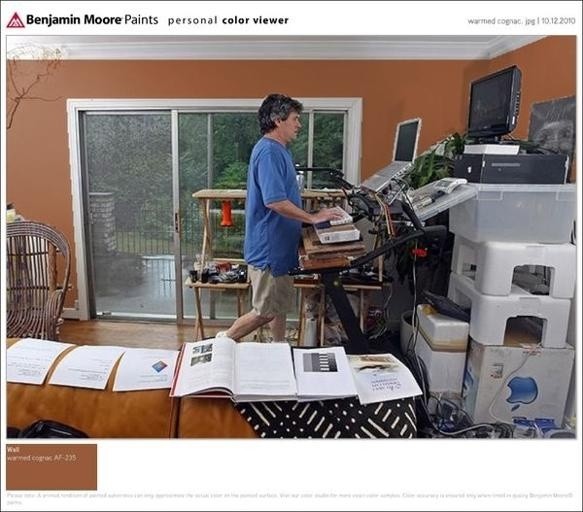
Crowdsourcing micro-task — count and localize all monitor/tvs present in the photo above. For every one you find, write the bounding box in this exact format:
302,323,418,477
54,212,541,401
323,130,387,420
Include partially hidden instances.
466,64,522,142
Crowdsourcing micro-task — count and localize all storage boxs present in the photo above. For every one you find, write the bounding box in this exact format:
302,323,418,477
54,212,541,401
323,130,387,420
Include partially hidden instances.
448,184,578,244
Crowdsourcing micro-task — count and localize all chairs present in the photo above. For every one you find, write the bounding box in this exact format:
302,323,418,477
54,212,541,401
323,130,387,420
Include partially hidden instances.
7,221,72,340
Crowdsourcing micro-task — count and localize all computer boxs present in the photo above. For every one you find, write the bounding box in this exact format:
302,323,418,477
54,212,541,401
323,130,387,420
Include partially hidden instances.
452,153,570,185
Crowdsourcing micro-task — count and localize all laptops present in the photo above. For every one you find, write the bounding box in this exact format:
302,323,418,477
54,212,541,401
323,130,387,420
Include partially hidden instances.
360,118,422,192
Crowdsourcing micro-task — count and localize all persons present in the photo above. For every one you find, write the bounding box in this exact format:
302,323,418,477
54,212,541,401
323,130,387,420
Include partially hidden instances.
215,93,345,343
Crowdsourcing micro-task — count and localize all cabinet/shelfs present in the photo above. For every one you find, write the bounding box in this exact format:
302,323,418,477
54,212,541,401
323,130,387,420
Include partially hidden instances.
187,186,251,345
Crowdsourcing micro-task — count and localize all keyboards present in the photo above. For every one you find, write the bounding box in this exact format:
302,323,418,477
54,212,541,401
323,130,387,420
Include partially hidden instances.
328,206,353,226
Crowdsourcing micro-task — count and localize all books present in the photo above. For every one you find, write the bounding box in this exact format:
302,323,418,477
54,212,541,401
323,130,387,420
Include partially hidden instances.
297,348,353,405
169,336,297,405
